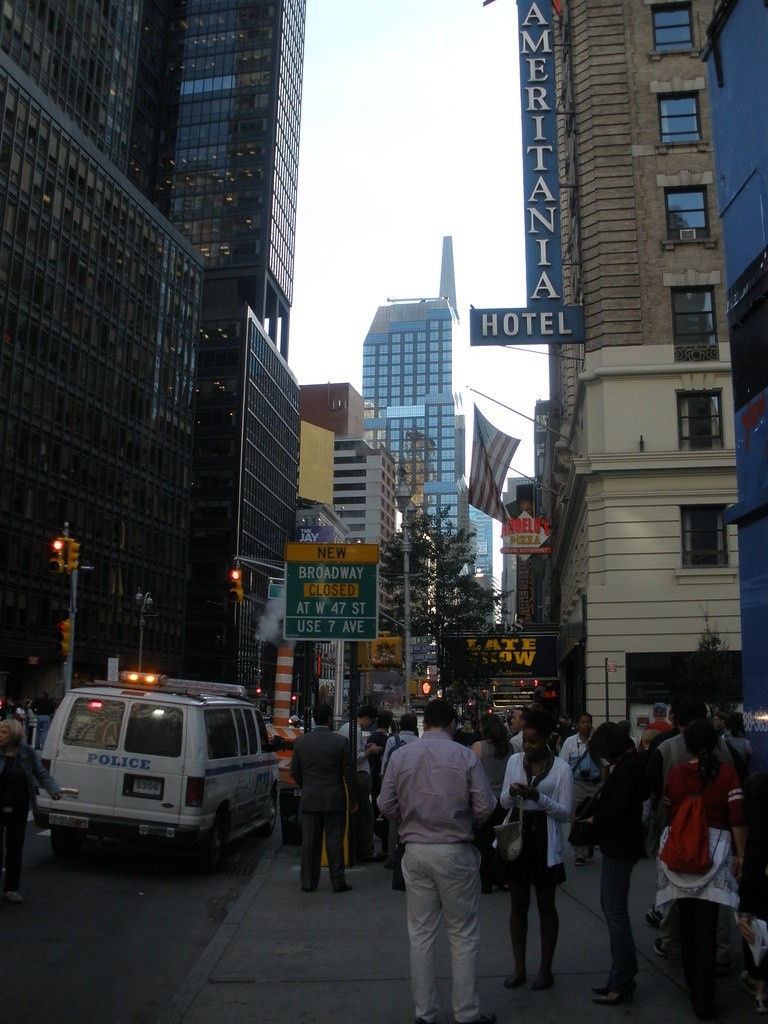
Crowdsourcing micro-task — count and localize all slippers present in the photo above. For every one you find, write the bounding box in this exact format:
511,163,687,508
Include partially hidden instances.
531,974,555,990
505,970,527,988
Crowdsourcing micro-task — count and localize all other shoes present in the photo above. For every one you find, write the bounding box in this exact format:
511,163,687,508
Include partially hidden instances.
645,912,660,928
364,852,384,863
574,858,585,865
333,882,352,892
592,991,636,1005
302,883,314,892
455,1013,497,1024
385,855,398,869
591,985,609,996
6,891,23,902
415,1017,436,1024
653,938,669,954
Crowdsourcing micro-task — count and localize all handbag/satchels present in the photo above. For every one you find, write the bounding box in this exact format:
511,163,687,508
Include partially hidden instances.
566,794,599,842
493,795,523,861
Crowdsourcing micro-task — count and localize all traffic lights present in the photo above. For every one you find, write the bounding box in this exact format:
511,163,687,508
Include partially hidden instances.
255,686,262,696
66,541,82,573
291,694,298,704
421,681,437,696
57,619,72,657
229,570,243,603
48,539,66,574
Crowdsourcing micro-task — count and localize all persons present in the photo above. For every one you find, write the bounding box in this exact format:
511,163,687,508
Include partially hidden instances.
0,689,60,750
733,772,768,1013
0,718,63,903
471,713,513,892
339,705,389,861
504,706,530,753
380,713,418,868
499,712,573,990
556,714,571,749
639,701,752,772
647,696,746,956
658,720,749,1020
289,704,353,893
590,721,651,1005
366,710,392,827
453,717,482,747
558,712,609,865
376,699,498,1024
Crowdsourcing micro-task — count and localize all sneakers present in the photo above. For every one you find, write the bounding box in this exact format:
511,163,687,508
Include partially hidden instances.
740,971,758,994
755,994,768,1012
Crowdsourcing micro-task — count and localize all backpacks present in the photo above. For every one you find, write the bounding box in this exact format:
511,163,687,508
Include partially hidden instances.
660,764,728,874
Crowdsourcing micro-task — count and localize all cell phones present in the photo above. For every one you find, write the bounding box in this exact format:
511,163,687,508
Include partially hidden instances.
513,783,525,790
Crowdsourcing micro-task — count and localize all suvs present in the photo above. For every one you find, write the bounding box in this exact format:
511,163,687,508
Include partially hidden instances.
32,670,288,877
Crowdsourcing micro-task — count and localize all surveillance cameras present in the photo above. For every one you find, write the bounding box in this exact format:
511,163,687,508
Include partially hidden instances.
145,598,153,609
135,594,143,606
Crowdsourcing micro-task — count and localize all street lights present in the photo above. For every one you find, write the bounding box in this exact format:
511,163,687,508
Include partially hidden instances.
252,628,266,708
134,591,154,672
392,479,417,712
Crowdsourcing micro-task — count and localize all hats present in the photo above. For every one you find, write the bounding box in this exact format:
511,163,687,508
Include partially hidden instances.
653,702,667,713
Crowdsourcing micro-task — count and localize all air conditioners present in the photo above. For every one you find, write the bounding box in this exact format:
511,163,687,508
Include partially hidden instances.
680,229,696,239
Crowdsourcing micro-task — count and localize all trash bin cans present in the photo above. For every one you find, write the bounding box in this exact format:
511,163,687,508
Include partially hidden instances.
280,786,301,841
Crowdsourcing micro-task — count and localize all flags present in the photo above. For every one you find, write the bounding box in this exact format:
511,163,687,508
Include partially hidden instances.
466,406,520,524
